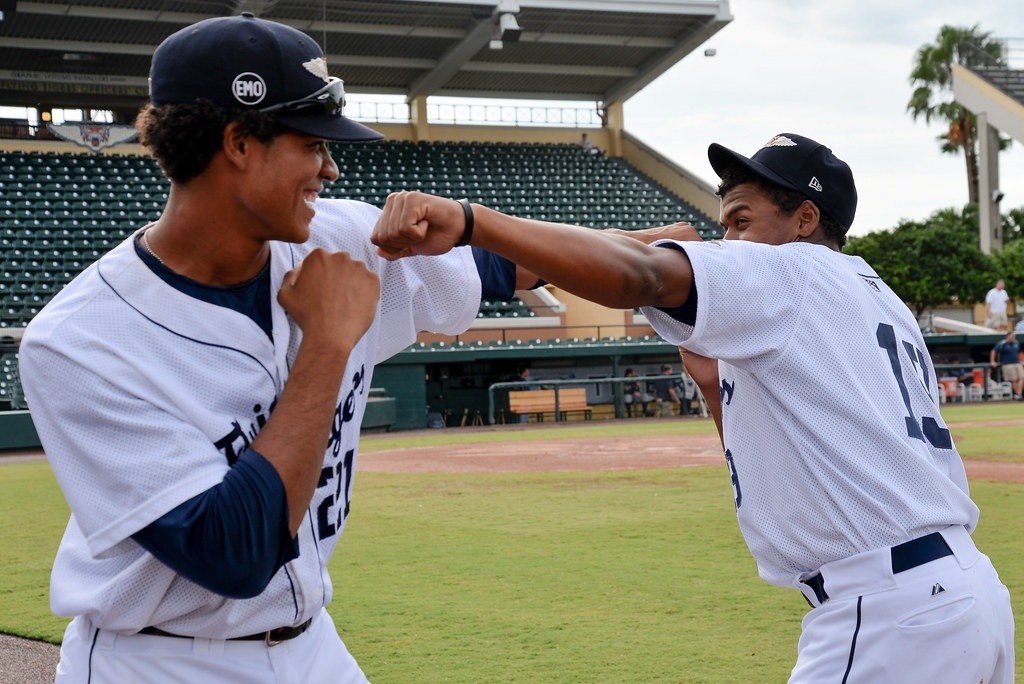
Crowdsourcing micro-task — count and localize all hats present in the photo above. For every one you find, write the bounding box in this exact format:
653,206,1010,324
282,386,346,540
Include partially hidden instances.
708,133,857,233
149,12,385,143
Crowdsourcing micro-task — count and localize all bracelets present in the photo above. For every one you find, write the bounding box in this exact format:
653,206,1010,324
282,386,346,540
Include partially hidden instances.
454,198,475,246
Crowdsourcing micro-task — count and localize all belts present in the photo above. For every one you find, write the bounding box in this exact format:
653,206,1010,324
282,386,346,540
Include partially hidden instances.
138,616,312,647
800,533,954,609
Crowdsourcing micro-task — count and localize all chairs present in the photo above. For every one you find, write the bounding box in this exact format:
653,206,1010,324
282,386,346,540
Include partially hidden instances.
0,139,727,410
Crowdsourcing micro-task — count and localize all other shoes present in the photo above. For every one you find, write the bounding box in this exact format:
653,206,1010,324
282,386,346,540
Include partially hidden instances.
1014,395,1022,401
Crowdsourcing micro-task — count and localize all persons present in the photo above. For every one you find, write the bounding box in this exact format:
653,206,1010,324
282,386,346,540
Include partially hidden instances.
990,332,1024,401
678,364,696,414
948,356,975,385
18,12,702,684
985,280,1009,331
513,368,531,423
624,368,653,417
656,366,680,416
371,133,1015,684
580,133,591,153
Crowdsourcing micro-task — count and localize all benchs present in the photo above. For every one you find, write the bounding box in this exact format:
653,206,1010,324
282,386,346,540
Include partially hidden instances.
938,383,966,404
969,380,1016,402
509,388,592,423
